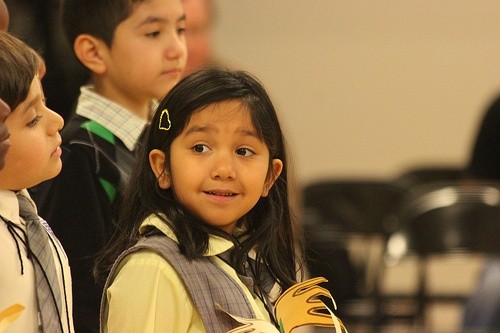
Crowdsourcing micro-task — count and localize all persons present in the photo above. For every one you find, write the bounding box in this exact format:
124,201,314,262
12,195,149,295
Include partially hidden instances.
92,64,353,333
0,26,80,333
38,0,191,253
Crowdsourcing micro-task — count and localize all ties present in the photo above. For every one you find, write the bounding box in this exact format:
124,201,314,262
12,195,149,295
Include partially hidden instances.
15,194,69,333
